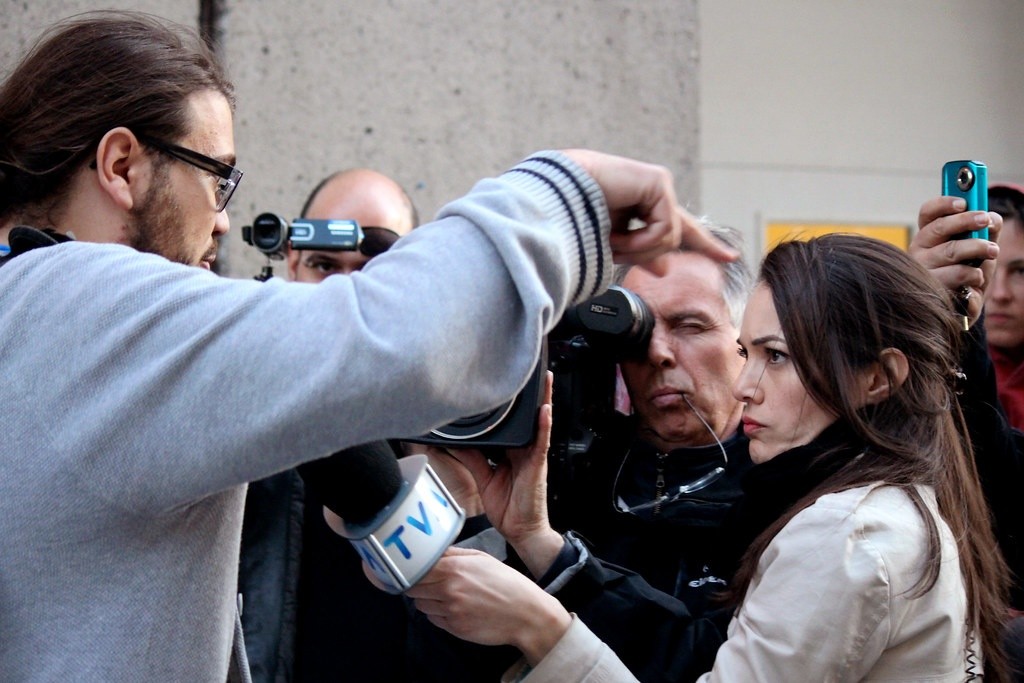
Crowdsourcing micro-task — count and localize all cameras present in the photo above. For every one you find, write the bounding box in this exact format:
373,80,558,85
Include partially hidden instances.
243,211,365,260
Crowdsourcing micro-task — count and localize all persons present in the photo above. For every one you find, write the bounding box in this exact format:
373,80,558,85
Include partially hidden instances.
0,9,739,683
229,165,1024,683
400,232,1008,683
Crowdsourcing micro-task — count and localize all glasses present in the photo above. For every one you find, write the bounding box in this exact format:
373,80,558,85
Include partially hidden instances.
89,134,243,213
610,393,729,519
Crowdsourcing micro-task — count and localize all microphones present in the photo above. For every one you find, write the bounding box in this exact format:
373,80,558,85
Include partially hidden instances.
318,438,467,594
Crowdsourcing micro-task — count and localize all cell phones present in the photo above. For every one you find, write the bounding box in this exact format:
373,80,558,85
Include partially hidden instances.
942,160,989,269
